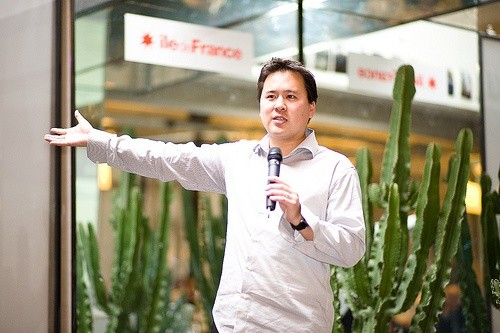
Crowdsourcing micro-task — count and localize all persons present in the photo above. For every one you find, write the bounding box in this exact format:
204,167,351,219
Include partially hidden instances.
44,57,366,333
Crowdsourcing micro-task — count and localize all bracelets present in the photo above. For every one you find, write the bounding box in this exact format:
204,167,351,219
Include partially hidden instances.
288,219,309,231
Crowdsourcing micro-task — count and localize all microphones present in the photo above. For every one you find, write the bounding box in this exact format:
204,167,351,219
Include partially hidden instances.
266,147,283,219
